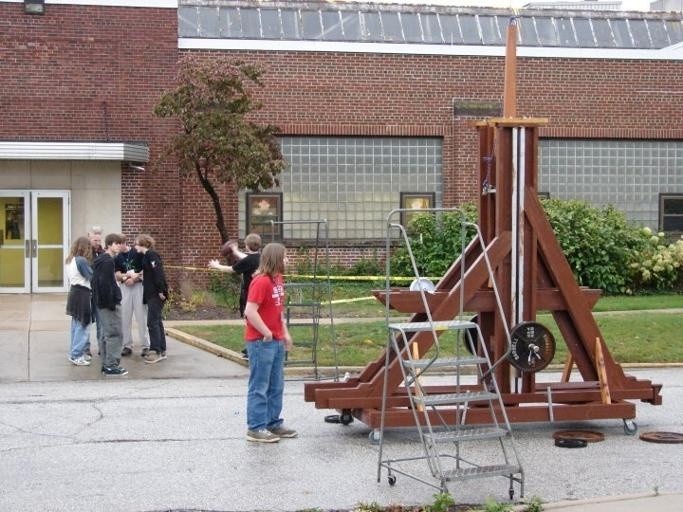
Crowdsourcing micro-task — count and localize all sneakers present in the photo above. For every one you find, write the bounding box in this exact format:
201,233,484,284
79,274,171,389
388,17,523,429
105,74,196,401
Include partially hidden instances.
104,367,128,376
68,347,167,366
245,429,280,442
270,427,298,438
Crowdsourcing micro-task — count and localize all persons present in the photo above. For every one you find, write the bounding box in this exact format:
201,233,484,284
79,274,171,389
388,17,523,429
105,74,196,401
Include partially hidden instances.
243,242,297,444
206,233,263,359
61,225,169,378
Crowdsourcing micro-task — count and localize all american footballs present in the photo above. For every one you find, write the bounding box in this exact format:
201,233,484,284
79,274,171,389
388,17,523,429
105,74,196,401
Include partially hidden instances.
221,239,239,256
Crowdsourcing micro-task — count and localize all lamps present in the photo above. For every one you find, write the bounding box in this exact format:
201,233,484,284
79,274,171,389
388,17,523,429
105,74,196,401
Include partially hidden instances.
23,0,45,15
